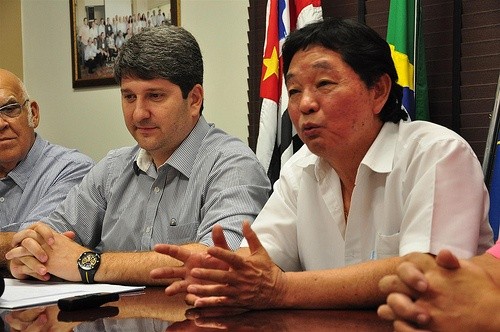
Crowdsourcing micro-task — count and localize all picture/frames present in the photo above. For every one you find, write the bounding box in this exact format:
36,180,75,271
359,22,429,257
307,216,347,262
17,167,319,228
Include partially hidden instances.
68,0,182,90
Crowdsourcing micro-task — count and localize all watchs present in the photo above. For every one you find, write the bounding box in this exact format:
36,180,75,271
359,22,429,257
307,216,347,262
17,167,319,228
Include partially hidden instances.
77,251,105,284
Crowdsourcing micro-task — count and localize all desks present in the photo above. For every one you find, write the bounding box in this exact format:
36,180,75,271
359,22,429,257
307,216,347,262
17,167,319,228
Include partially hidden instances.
0,280,393,332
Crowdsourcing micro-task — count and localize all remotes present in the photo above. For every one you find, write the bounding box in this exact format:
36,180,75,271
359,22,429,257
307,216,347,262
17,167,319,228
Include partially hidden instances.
58,291,119,312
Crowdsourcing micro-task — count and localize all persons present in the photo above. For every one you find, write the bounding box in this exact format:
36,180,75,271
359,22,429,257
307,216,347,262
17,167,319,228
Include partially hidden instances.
376,226,500,332
0,67,95,268
5,25,272,287
75,9,167,74
150,16,495,320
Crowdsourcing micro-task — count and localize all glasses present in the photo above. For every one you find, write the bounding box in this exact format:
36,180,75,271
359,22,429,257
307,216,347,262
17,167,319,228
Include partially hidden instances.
0,99,29,117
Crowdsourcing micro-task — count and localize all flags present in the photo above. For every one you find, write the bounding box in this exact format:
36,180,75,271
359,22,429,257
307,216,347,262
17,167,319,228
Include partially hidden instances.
255,0,325,185
385,0,431,121
